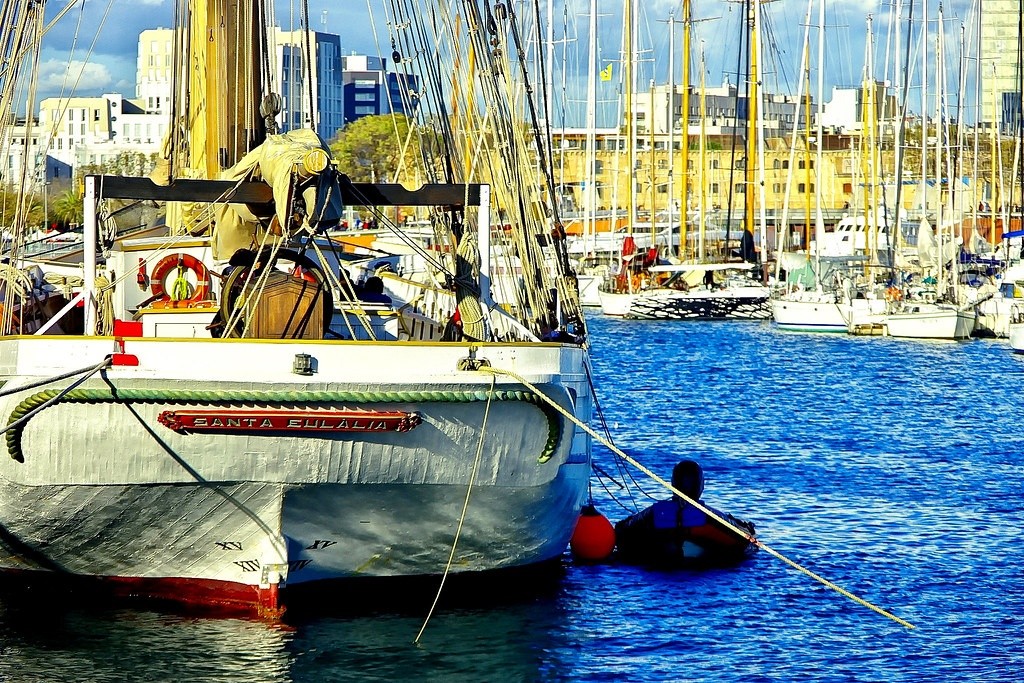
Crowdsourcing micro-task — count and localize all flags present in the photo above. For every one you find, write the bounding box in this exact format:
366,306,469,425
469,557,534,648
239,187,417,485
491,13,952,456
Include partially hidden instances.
599,63,612,81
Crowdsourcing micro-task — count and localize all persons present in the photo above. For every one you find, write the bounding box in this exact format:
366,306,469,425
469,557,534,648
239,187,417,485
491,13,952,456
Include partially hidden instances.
358,277,391,303
340,268,361,300
615,460,757,540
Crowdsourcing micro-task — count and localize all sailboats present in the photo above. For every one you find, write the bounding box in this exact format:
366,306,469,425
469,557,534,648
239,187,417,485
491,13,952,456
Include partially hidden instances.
357,0,1023,355
0,0,921,639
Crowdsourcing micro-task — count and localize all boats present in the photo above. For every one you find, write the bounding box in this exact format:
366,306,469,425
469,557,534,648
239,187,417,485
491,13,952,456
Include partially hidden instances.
615,458,754,570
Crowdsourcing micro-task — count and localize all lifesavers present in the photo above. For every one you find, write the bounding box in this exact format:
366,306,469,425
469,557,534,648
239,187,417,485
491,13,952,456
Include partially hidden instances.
151,253,211,301
